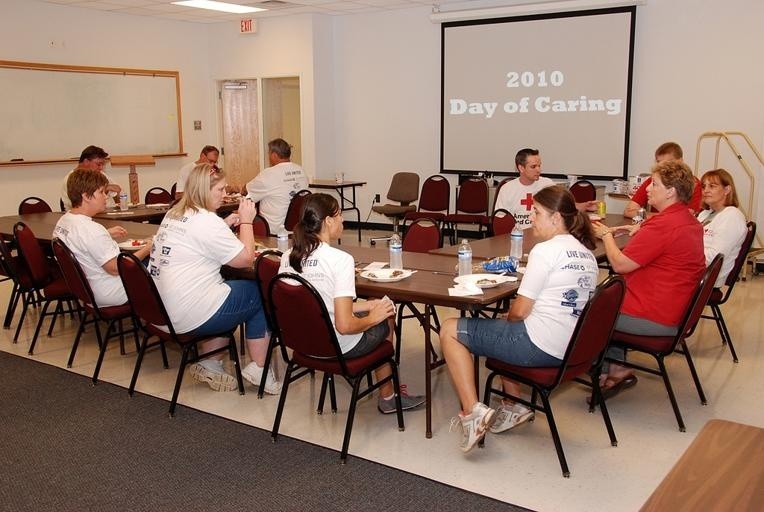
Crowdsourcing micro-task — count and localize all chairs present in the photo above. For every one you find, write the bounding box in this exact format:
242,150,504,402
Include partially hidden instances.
708,220,756,363
401,175,450,247
587,253,724,433
477,274,626,479
489,209,517,236
402,218,441,254
370,172,419,246
116,251,245,417
569,181,597,202
145,187,172,204
0,234,33,329
443,176,489,245
253,249,340,416
13,222,103,355
235,215,270,237
19,196,52,215
50,236,169,384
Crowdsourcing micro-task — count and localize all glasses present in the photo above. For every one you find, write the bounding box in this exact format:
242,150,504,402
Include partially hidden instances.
209,164,220,175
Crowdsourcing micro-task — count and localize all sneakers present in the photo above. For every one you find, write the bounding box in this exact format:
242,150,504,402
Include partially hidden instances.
189,359,238,392
449,402,496,453
489,402,535,435
377,384,426,413
241,360,281,394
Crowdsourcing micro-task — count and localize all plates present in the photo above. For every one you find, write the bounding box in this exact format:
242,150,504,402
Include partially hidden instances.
360,269,411,283
117,242,146,250
146,204,170,208
255,251,269,260
506,276,518,282
453,274,507,288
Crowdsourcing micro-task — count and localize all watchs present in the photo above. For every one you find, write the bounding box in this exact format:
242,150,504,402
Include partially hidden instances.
600,230,612,239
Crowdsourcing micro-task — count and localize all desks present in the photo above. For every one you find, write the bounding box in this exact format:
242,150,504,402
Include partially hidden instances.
639,419,764,512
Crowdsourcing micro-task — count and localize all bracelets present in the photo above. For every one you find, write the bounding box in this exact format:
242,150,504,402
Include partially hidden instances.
240,223,253,224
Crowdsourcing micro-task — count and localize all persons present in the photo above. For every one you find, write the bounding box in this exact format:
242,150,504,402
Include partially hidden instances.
175,146,218,201
242,139,309,238
624,143,701,220
586,160,705,406
494,149,599,230
53,169,152,317
61,145,122,213
695,170,746,295
277,194,425,414
439,186,599,453
146,164,282,395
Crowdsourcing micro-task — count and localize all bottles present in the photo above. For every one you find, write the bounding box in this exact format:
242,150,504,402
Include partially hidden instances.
388,234,404,273
277,223,289,252
457,238,473,278
510,222,524,259
596,201,607,219
119,187,129,211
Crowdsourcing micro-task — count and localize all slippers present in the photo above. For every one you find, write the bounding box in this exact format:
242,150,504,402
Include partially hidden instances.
585,389,617,406
604,373,637,397
